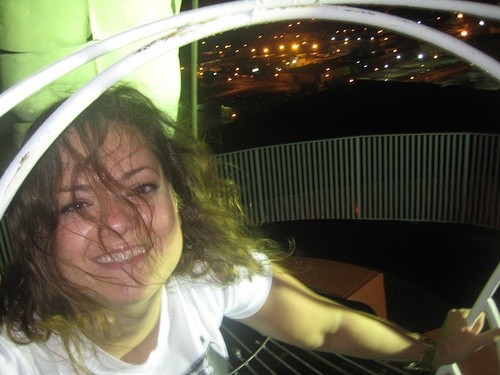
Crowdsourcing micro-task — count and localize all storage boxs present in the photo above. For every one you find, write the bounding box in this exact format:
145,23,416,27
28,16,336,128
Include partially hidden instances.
278,254,386,320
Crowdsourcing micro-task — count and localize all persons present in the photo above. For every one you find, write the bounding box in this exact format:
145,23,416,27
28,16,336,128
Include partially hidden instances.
0,85,500,375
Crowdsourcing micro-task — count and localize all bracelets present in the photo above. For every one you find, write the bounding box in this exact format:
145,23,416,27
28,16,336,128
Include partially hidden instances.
414,338,435,367
